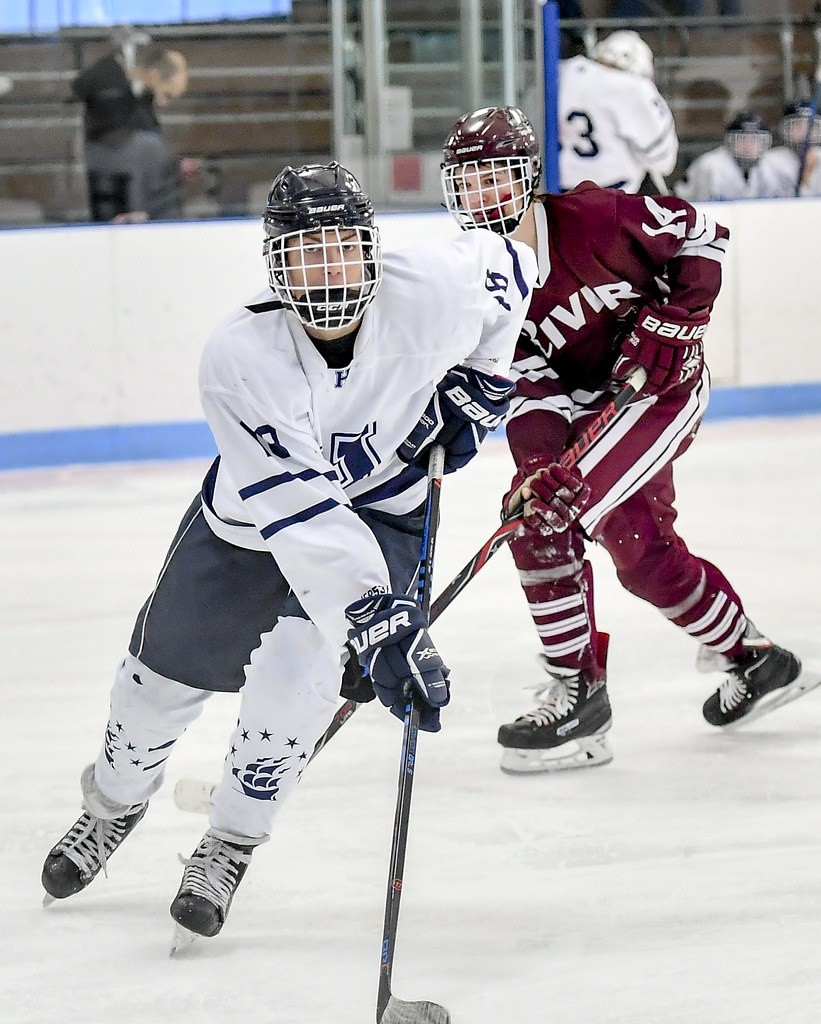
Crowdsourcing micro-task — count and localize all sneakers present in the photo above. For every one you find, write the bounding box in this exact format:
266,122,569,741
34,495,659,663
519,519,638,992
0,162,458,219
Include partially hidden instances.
168,828,271,959
41,760,149,909
702,619,821,730
497,632,613,774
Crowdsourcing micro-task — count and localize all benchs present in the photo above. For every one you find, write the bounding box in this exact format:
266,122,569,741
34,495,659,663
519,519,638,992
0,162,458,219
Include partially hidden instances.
0,0,821,230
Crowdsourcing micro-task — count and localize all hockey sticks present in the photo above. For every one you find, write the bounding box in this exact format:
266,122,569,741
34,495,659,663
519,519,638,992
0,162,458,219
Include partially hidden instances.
374,443,457,1024
171,367,650,819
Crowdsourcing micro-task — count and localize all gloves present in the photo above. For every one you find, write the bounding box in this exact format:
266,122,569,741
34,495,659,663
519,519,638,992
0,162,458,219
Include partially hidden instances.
344,594,451,733
502,452,592,537
606,300,711,405
396,364,516,476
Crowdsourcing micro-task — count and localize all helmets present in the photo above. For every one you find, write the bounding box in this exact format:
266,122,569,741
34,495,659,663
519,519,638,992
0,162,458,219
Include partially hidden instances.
262,161,383,331
440,106,542,237
781,98,821,155
724,113,773,170
595,28,654,81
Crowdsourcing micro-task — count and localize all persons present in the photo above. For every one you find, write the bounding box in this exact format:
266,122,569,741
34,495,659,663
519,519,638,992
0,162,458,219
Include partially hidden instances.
68,50,190,224
39,161,537,956
516,31,679,198
674,100,821,202
440,107,821,776
